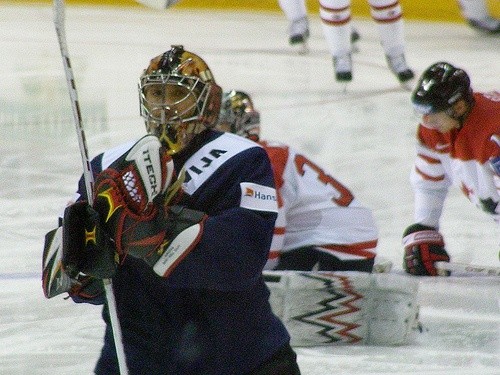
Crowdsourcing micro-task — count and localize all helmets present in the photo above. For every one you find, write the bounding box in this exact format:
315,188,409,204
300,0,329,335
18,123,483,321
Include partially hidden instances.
219,89,261,142
138,44,222,130
409,61,471,115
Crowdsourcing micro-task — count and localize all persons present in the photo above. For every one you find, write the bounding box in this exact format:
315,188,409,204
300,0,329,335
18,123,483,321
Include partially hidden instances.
277,0,500,81
213,89,378,271
40,43,303,375
399,61,500,278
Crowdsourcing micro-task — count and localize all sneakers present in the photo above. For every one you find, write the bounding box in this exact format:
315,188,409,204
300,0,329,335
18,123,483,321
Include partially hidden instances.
468,16,500,34
386,55,416,90
331,49,352,93
289,16,312,56
350,25,361,54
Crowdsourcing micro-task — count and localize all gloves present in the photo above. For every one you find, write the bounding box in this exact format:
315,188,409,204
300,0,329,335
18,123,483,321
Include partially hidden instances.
400,224,452,278
91,168,208,280
42,201,114,300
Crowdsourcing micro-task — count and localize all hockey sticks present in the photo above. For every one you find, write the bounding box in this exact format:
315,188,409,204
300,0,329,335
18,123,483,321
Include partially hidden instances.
51,0,128,375
434,261,500,276
